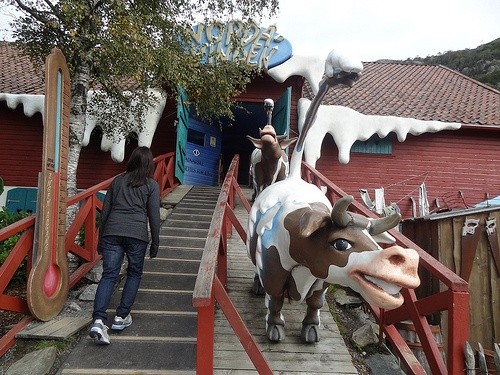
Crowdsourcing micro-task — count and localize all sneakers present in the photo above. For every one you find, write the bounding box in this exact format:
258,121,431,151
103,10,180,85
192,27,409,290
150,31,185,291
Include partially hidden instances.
111,312,132,329
88,319,110,344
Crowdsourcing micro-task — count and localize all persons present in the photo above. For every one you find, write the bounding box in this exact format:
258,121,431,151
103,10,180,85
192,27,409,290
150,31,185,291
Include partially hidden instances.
89,146,160,345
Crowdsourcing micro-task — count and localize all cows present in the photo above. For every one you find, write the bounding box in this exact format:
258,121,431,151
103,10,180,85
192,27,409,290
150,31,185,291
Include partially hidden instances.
246,71,421,343
246,106,299,202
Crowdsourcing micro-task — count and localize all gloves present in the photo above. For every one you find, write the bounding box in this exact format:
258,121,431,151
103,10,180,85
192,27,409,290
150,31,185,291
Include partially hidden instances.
150,243,158,258
97,239,104,255
